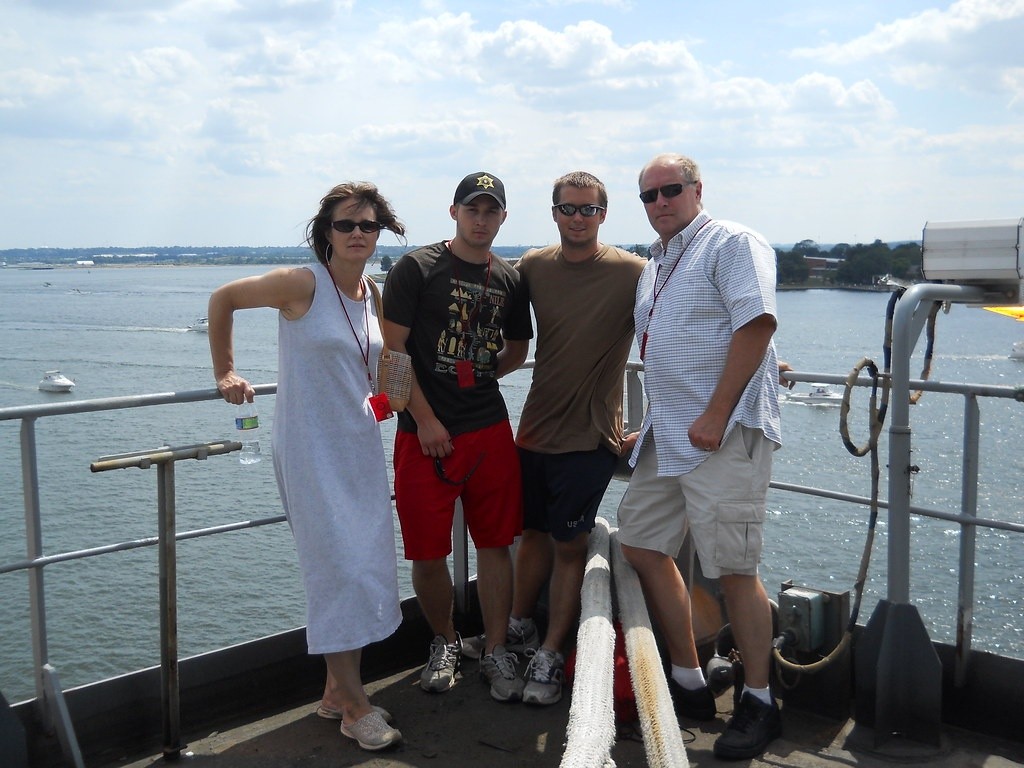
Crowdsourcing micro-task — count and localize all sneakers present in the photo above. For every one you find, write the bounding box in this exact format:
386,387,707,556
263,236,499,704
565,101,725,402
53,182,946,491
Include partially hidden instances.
419,630,466,693
524,646,567,704
457,617,541,659
477,644,526,701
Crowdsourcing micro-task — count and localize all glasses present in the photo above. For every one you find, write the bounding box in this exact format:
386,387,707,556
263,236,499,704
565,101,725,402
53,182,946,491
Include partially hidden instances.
638,183,682,204
326,220,383,233
552,202,606,217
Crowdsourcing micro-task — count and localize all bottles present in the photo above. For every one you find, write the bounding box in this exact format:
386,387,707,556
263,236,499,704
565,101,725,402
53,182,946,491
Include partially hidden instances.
234,393,262,464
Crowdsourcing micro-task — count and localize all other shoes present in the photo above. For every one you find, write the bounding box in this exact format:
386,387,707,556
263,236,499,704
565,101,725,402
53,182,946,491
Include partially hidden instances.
658,679,719,721
709,689,783,760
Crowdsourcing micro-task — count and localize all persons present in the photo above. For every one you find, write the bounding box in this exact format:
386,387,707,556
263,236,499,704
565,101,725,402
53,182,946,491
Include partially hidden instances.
615,154,784,761
474,170,796,705
208,178,407,749
380,171,534,702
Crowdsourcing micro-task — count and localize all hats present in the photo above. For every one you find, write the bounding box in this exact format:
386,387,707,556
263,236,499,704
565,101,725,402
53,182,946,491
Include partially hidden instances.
453,172,506,210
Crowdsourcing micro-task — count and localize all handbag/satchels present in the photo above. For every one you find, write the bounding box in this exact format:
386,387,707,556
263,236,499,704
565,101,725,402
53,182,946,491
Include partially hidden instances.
375,349,413,413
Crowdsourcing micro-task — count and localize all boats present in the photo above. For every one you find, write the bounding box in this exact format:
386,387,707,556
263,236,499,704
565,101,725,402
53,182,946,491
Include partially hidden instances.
188,316,209,332
38,371,76,392
784,383,843,406
1009,340,1023,359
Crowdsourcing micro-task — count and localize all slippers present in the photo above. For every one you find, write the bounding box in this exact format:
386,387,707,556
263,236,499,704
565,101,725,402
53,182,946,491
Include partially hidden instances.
341,712,401,751
315,701,394,725
